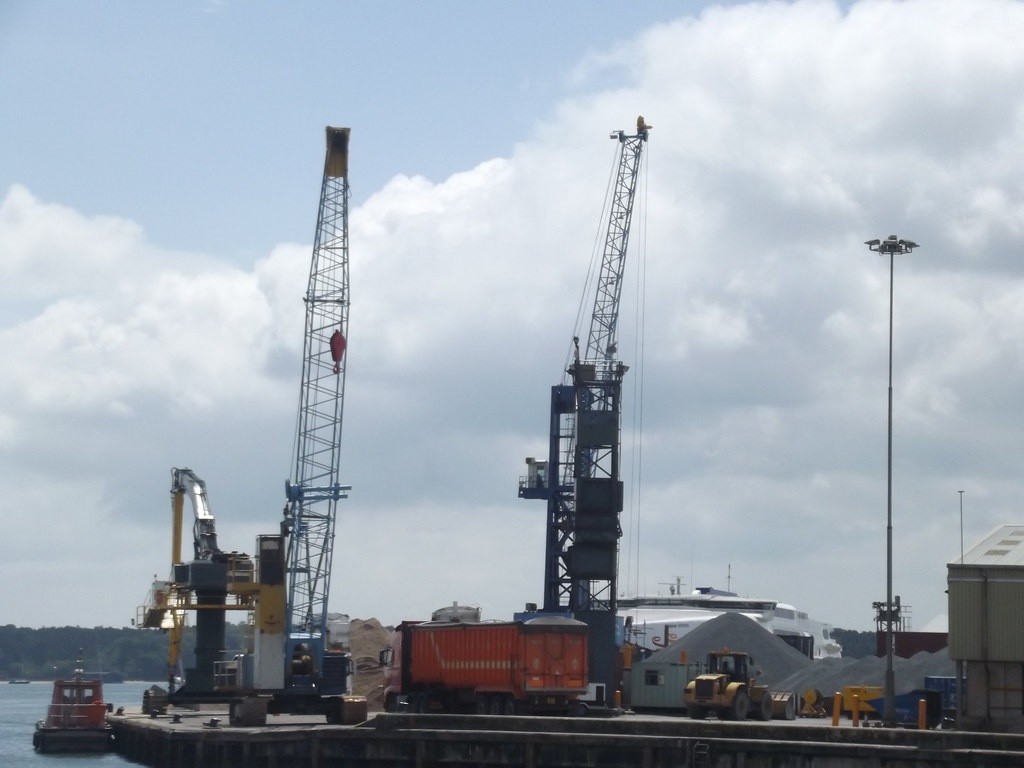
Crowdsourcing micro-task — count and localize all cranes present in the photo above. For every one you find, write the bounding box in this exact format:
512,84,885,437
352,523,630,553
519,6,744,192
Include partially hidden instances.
273,123,354,710
517,114,660,712
126,464,262,701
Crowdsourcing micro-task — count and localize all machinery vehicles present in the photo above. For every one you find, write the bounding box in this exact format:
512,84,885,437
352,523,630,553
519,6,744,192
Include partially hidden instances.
684,649,797,720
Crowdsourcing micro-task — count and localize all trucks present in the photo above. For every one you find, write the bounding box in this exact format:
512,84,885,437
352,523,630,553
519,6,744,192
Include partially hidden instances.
380,618,589,716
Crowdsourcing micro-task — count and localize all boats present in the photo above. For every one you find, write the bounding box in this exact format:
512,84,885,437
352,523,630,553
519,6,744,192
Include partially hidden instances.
29,643,115,750
